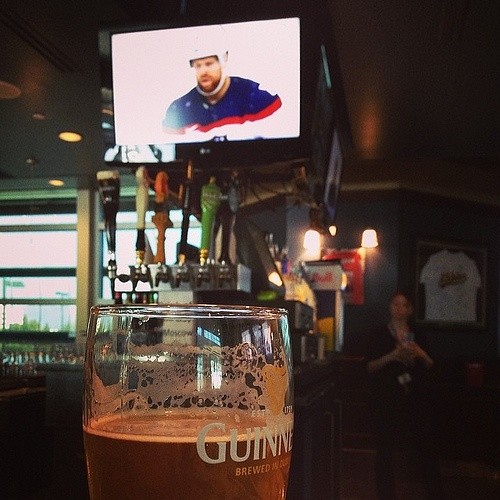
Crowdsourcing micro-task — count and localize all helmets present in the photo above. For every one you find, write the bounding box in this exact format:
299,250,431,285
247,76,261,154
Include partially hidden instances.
187,31,228,95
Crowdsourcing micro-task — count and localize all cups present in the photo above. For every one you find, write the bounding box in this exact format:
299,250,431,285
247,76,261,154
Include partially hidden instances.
81,303,296,500
317,338,327,360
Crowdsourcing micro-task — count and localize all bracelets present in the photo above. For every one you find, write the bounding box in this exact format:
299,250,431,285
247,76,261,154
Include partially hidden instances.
423,352,429,360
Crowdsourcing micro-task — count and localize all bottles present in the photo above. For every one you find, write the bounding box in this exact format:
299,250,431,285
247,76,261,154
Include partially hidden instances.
114,292,159,305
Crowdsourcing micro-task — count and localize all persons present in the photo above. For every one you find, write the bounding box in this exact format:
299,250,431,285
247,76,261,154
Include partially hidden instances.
362,290,444,500
159,37,282,142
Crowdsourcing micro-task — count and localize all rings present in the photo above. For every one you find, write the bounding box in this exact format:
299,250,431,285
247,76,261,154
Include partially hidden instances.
413,348,417,352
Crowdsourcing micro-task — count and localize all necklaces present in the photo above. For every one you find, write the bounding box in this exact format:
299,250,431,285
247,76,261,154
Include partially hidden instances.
390,320,397,337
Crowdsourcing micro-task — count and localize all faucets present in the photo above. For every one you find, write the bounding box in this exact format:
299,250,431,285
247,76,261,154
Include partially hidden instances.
218,259,233,289
105,259,130,291
195,260,211,289
175,262,190,290
133,264,151,287
155,261,170,287
